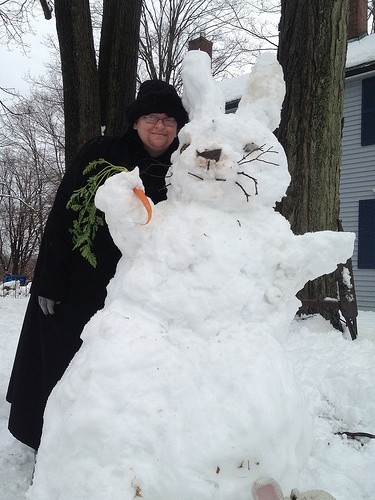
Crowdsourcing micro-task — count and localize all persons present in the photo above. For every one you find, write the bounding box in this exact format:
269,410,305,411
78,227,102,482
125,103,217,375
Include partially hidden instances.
6,80,189,485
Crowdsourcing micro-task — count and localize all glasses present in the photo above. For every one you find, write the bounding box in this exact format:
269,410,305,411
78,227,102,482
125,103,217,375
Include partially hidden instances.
140,115,178,128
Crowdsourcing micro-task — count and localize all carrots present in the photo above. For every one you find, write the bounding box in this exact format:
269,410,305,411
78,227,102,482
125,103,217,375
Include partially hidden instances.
133,187,152,225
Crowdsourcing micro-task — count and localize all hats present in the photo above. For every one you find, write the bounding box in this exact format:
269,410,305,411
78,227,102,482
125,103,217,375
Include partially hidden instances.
127,80,188,133
